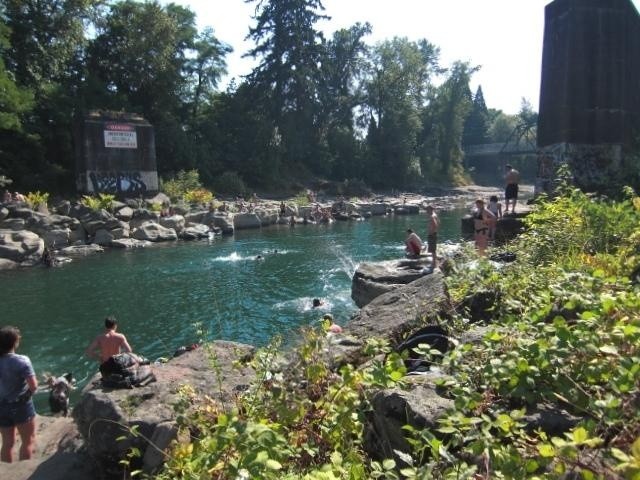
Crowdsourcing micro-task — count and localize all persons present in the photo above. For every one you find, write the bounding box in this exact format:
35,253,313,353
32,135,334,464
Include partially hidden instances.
207,202,254,217
474,200,494,256
426,206,440,267
313,298,326,306
50,378,69,417
324,314,342,332
280,191,329,226
0,326,38,463
3,189,24,202
487,196,502,220
504,164,520,212
87,316,132,363
406,229,421,256
